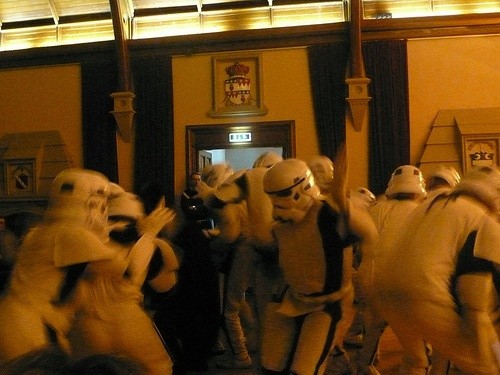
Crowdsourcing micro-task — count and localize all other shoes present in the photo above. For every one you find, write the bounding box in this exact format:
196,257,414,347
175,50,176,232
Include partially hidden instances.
214,355,252,370
343,333,364,349
358,364,381,375
331,346,353,373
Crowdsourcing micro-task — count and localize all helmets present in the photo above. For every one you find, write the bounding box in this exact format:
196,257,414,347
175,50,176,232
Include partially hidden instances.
385,164,428,199
44,167,109,243
431,164,460,189
307,155,334,186
253,152,284,169
262,158,322,225
202,164,234,188
110,190,143,221
461,166,500,215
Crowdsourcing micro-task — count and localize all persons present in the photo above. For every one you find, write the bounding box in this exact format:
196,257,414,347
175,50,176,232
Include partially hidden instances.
0,166,179,375
195,151,500,375
179,171,206,227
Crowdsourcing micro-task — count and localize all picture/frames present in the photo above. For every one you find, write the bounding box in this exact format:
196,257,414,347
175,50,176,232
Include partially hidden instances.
206,52,269,119
3,157,37,199
461,133,500,176
